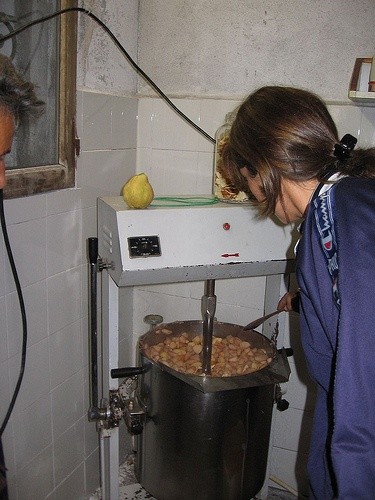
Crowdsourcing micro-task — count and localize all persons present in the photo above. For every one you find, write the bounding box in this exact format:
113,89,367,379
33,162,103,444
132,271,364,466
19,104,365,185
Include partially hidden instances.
0,51,46,195
221,86,374,500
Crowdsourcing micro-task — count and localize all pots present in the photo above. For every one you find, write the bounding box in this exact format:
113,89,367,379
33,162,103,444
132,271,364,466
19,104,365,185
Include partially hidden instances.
125,320,291,500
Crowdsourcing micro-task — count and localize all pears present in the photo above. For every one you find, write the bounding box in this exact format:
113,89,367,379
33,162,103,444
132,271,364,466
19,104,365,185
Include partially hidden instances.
123,172,153,209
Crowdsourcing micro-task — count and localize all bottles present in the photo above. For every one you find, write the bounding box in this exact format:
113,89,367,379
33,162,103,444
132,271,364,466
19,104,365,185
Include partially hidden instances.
213,112,257,201
